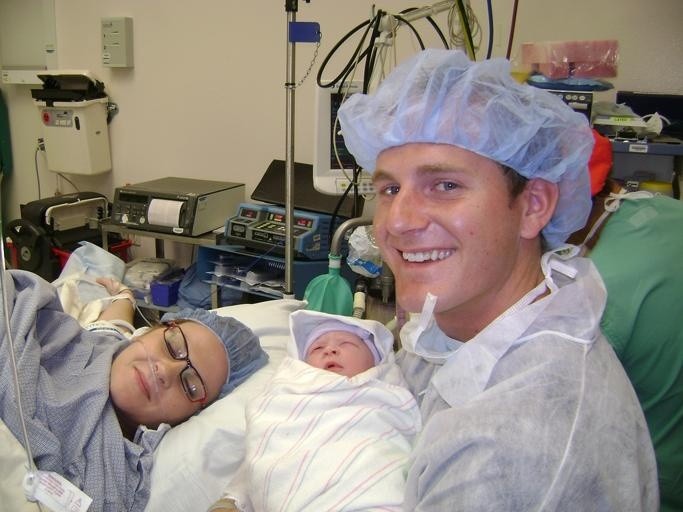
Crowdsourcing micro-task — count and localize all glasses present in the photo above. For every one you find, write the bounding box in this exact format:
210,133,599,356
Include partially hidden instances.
164,322,207,409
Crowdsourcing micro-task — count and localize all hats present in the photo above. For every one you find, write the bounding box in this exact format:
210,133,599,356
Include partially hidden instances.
159,306,269,397
590,127,611,197
336,46,594,254
303,320,380,366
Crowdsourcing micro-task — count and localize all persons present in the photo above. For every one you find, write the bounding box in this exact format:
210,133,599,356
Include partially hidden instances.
204,46,661,511
564,129,683,510
0,268,270,512
245,309,424,512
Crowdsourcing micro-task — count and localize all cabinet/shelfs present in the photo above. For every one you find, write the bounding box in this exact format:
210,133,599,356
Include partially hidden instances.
102,218,224,318
